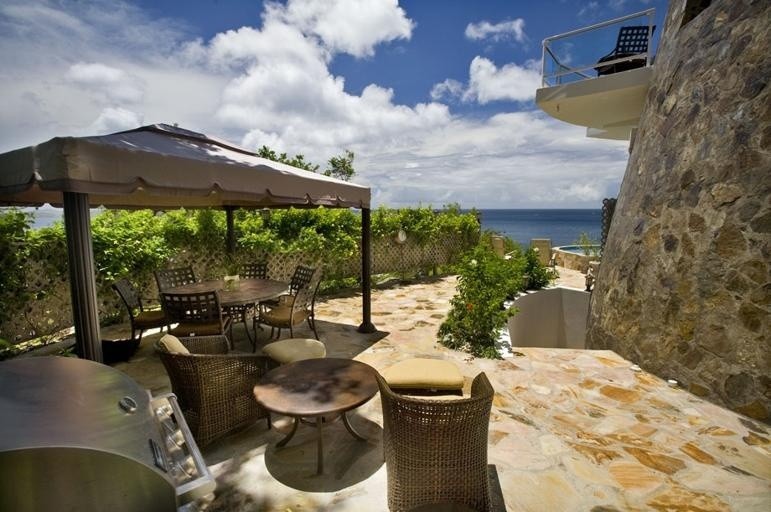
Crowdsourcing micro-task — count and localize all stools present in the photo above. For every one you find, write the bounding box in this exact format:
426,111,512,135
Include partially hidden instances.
379,357,464,392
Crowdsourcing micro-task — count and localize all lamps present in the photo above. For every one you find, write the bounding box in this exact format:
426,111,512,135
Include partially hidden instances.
262,207,270,227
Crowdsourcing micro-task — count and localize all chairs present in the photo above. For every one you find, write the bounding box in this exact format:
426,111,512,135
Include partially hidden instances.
593,25,656,76
490,235,512,261
374,369,494,512
111,263,323,452
530,239,559,275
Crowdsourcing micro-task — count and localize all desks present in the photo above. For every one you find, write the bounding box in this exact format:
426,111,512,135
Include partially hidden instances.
253,357,380,476
